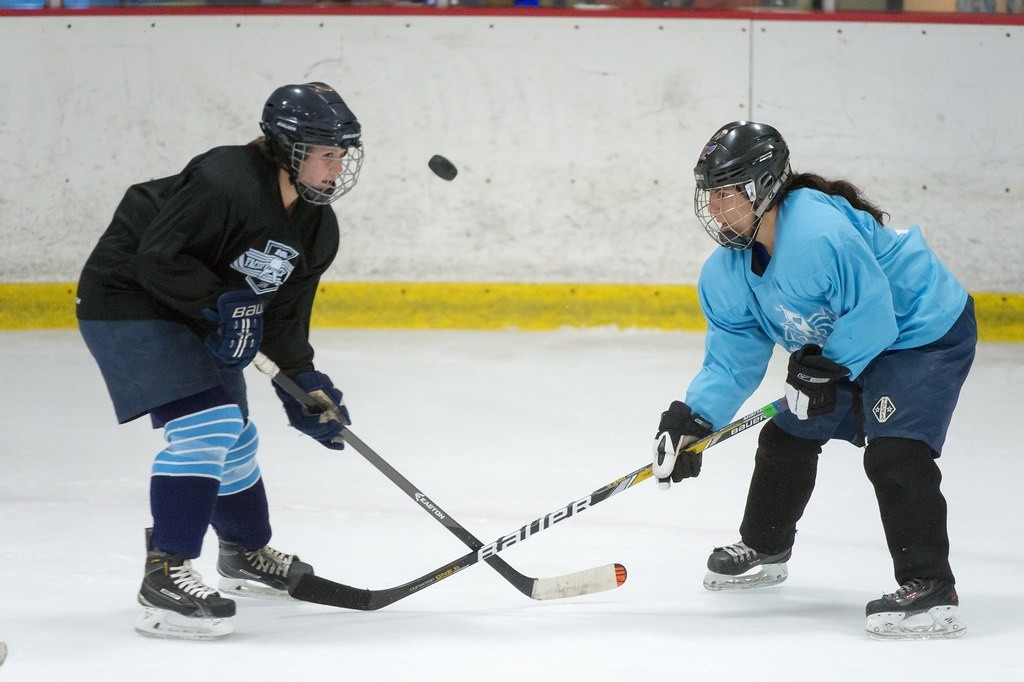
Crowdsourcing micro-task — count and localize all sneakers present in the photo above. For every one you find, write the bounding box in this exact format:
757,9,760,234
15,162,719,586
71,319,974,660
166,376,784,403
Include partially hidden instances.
865,577,971,640
215,539,313,599
136,547,236,639
704,543,792,590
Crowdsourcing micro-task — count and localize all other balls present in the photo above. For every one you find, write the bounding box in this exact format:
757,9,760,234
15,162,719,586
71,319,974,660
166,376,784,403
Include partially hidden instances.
427,154,457,183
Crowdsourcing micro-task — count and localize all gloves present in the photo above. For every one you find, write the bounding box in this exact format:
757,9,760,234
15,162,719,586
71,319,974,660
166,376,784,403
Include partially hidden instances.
654,400,711,488
787,342,843,419
206,288,264,375
278,370,351,450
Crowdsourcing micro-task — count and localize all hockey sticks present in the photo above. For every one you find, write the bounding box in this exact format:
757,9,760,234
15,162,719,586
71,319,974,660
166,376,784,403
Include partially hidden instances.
193,307,629,600
287,397,789,611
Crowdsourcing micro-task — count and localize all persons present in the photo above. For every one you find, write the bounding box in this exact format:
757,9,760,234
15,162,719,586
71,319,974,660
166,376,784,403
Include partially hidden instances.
653,122,980,637
76,81,364,642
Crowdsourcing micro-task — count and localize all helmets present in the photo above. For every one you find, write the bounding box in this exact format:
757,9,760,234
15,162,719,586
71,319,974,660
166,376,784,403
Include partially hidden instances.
695,121,791,251
259,82,365,206
